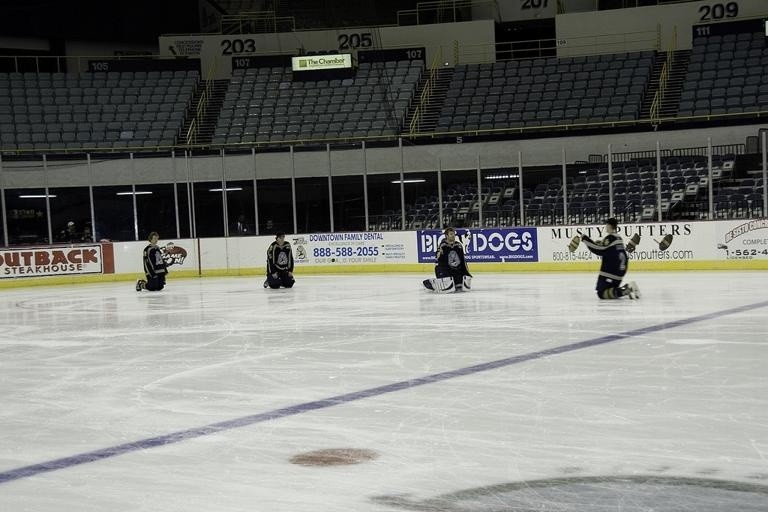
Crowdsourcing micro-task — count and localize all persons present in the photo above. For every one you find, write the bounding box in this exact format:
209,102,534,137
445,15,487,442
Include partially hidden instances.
263,231,294,289
582,217,632,299
230,215,248,235
134,231,168,291
59,221,79,242
260,219,275,235
435,226,470,290
79,221,101,242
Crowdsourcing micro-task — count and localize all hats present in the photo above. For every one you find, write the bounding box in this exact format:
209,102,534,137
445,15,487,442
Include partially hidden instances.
606,218,618,225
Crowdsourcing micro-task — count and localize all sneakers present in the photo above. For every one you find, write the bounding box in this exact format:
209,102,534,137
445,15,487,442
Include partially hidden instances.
625,283,639,299
263,282,268,288
136,280,146,291
